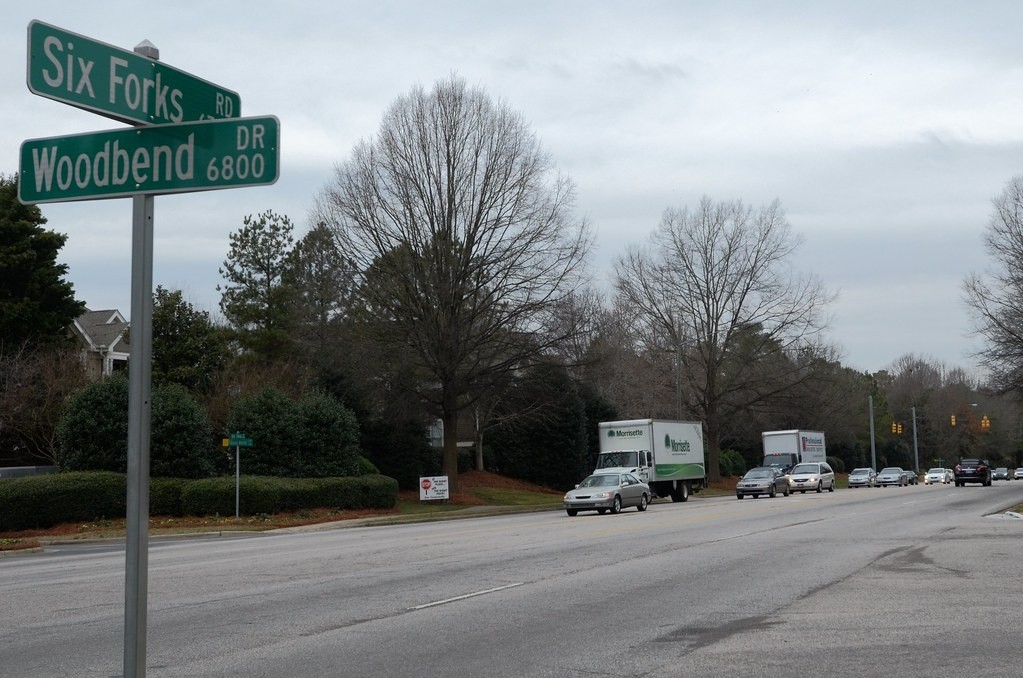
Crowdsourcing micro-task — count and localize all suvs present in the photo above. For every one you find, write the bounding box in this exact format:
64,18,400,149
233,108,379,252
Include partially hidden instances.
955,458,992,487
991,467,1023,481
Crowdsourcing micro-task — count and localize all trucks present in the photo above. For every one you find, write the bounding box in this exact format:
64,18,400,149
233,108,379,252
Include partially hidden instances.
761,429,826,473
594,417,707,502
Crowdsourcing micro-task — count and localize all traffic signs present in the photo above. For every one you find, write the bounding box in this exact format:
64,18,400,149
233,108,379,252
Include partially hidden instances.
17,114,281,205
223,433,253,446
26,19,240,128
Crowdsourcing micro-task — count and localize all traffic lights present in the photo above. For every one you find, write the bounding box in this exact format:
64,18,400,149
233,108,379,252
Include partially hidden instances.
951,416,956,426
982,416,990,428
891,423,902,433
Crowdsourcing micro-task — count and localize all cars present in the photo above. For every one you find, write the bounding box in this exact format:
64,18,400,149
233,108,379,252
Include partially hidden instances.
735,466,789,499
848,468,877,488
924,468,954,485
789,462,835,493
904,471,919,485
876,467,908,487
563,473,650,516
